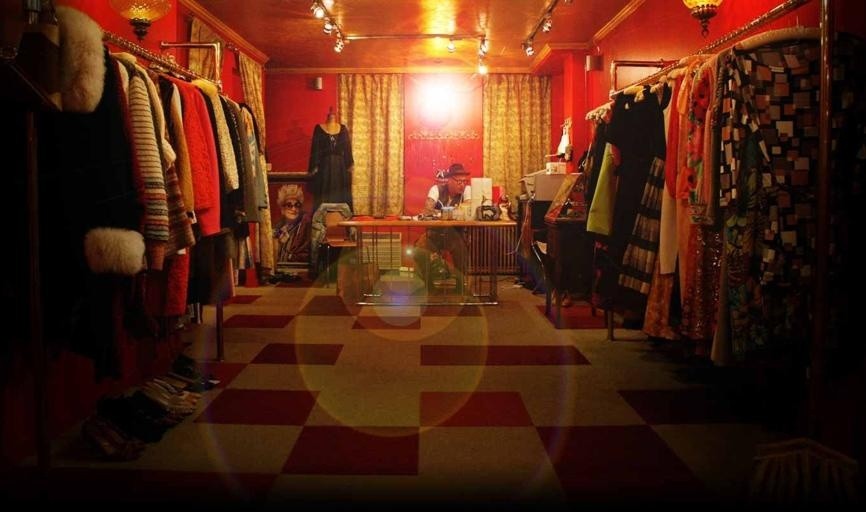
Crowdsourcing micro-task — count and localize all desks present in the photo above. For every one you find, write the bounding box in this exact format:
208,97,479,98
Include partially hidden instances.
337,220,518,306
545,215,598,331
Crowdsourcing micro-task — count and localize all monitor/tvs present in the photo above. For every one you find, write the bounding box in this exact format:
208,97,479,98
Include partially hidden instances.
471,178,492,218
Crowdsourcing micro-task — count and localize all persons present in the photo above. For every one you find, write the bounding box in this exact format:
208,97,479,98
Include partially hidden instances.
413,165,470,293
271,183,316,263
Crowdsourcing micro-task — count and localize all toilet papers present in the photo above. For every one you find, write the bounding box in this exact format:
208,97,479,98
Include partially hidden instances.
470,199,482,218
470,177,482,199
482,178,493,200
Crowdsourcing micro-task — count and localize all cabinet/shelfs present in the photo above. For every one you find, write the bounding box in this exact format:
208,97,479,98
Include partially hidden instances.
518,199,552,290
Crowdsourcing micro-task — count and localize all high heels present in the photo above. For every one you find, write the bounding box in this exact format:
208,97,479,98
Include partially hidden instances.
552,288,573,307
81,365,222,461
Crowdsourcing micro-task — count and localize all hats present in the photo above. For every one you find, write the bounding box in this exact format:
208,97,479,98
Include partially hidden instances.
445,163,470,176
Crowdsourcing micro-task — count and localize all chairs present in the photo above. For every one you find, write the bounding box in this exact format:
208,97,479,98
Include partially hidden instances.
318,203,372,287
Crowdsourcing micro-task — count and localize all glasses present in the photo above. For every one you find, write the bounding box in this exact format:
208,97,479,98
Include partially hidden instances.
450,176,469,185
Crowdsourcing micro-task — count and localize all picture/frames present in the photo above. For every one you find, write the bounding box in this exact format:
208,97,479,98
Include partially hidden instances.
267,173,310,270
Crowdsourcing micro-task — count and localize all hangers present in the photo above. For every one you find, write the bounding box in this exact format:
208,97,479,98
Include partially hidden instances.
104,33,223,91
580,1,836,122
